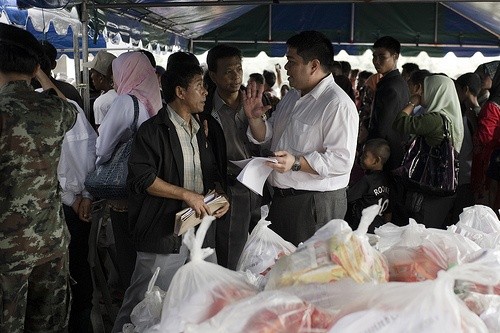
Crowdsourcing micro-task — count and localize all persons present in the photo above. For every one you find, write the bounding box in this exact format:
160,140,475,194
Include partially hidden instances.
240,30,359,245
0,22,500,333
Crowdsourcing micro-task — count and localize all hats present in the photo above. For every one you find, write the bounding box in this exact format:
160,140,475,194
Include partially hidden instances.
81,48,117,76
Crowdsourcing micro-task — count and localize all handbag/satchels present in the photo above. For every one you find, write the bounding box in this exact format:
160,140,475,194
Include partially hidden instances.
83,93,141,199
402,116,459,194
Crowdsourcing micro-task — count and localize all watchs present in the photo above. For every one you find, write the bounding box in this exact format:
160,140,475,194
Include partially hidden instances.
291,154,301,172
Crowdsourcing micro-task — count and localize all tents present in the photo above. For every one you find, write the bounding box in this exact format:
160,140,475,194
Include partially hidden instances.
0,0,499,121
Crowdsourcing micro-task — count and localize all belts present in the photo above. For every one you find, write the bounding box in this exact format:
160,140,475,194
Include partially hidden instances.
272,185,314,198
